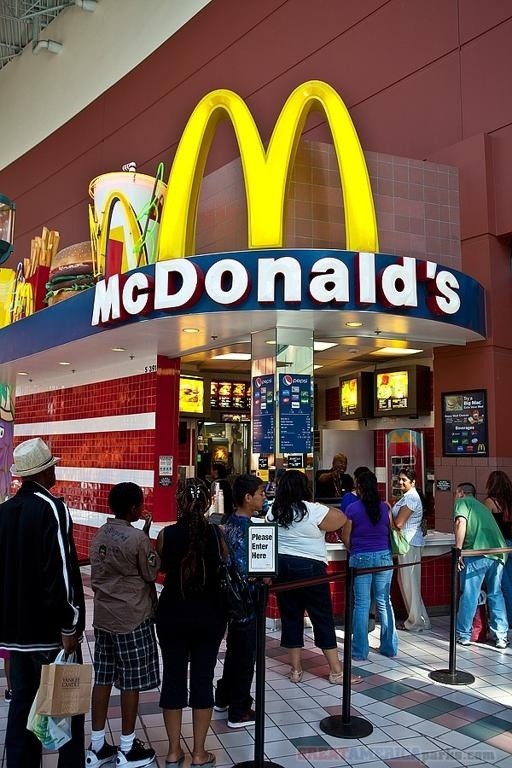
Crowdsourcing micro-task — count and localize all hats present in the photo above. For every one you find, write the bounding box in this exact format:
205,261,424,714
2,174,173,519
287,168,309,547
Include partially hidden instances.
10,437,62,477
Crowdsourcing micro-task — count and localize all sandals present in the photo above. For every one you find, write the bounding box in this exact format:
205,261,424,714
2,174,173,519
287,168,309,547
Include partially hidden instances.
328,670,364,686
288,667,304,683
396,623,408,630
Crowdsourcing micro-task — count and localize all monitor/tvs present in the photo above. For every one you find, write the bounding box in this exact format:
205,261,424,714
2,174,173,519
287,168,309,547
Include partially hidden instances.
373,364,433,419
338,371,374,421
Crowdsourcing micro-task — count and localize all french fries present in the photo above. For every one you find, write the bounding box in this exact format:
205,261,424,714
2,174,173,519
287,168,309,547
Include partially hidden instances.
23,226,61,279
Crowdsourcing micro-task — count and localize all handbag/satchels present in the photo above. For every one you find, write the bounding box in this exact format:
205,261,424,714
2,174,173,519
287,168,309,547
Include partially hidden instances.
35,649,93,717
420,518,428,537
470,589,492,643
208,522,255,610
385,500,410,555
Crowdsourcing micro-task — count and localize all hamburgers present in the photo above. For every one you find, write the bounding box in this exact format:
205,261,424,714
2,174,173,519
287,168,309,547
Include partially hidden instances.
42,241,98,307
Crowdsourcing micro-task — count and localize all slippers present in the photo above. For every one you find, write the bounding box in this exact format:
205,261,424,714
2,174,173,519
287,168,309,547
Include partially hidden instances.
190,750,216,768
164,750,185,768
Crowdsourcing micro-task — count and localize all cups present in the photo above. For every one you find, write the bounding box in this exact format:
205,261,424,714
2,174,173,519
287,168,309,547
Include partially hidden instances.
90,170,169,280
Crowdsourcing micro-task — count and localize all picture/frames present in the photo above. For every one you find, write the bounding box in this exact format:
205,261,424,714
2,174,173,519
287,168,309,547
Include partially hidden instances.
441,387,489,457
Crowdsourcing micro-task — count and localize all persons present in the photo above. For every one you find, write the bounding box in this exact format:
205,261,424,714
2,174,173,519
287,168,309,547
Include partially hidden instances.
155,478,232,768
205,451,433,728
1,436,88,768
85,481,164,768
0,649,13,703
451,481,512,648
479,470,512,642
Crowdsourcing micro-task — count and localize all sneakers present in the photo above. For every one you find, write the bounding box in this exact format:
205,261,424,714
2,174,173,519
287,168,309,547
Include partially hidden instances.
457,634,471,646
213,702,229,713
84,736,123,768
495,637,509,649
226,707,256,728
115,738,156,768
